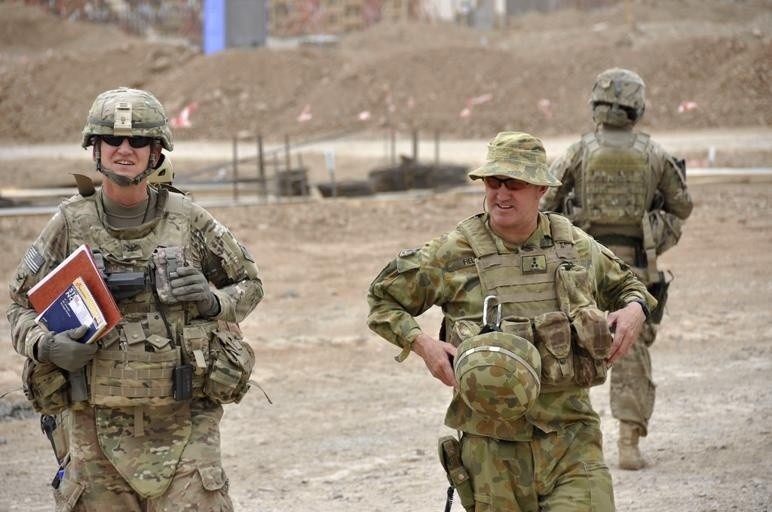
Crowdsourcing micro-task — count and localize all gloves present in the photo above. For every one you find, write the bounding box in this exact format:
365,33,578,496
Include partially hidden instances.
169,267,219,318
38,326,98,373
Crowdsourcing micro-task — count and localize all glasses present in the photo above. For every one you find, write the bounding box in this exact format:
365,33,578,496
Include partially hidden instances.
97,135,152,148
482,176,529,190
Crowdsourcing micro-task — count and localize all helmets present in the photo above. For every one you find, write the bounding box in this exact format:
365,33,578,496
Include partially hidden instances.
591,69,646,126
453,331,542,424
82,86,174,151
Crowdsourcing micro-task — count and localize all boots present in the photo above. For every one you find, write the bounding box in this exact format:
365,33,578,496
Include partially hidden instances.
617,421,645,469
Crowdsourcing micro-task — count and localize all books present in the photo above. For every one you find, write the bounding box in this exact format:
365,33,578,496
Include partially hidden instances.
27,244,123,344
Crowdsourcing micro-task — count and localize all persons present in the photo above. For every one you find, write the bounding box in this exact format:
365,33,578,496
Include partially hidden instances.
7,86,264,512
538,69,693,470
366,131,659,512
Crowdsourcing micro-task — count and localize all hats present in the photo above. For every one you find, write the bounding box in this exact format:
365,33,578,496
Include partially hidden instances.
468,131,563,187
